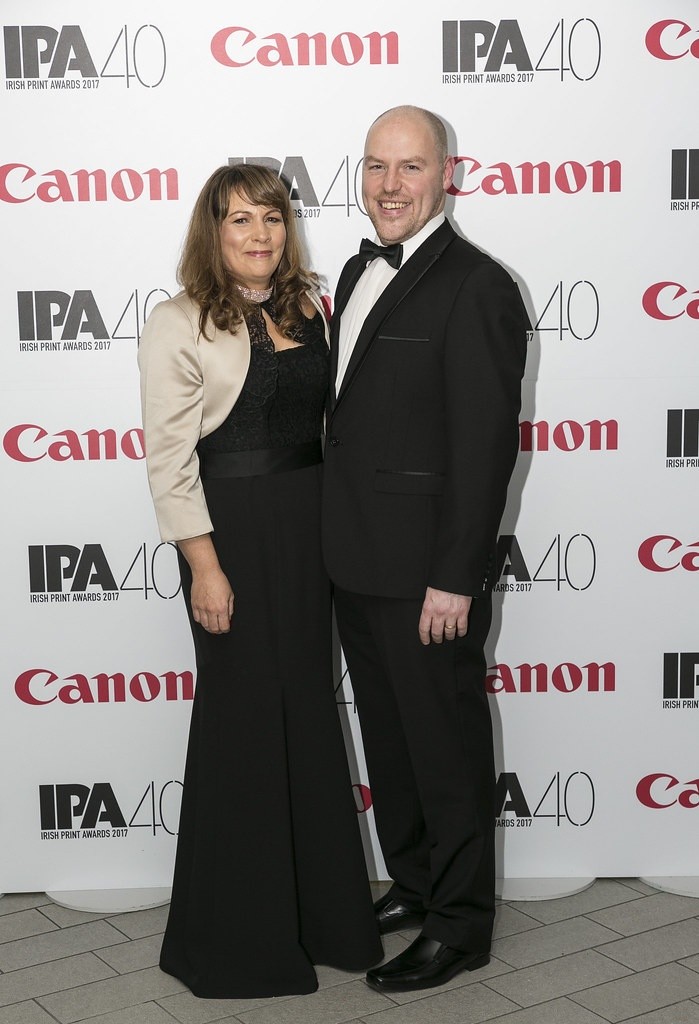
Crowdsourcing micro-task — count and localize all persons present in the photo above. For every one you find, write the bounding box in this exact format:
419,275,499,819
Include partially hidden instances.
319,106,528,991
137,164,386,1000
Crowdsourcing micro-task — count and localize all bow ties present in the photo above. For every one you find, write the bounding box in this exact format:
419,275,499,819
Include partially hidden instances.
359,238,403,270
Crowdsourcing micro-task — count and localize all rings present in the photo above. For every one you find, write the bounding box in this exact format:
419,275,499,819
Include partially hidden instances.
445,625,456,629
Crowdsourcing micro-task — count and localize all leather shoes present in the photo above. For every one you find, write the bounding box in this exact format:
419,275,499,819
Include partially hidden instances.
366,935,490,991
374,894,425,935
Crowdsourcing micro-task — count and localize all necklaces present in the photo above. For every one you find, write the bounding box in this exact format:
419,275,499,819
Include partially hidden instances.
236,285,273,302
